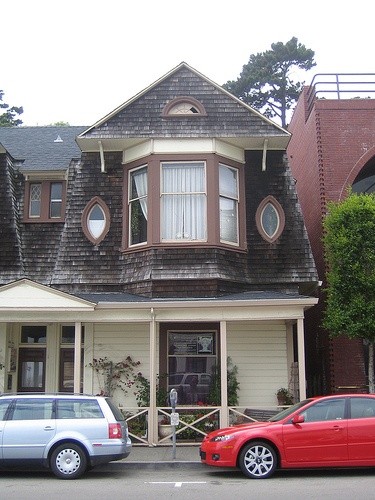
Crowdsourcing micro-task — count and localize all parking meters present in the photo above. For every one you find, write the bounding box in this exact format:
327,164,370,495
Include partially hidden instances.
169,388,180,459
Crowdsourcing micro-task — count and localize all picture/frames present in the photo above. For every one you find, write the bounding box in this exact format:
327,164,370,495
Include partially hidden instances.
196,335,213,355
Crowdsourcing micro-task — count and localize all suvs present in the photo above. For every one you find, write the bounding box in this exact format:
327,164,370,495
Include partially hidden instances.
168,371,213,399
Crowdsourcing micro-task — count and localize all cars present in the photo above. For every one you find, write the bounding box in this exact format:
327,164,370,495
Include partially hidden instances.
200,394,375,479
0,391,131,480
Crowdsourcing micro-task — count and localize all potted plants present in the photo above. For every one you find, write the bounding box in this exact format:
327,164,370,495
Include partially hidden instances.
208,356,240,425
275,388,294,410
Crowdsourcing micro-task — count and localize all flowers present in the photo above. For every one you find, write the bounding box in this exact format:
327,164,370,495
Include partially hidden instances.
85,356,215,439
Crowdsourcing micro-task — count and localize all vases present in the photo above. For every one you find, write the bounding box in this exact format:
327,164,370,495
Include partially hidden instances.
159,425,173,442
